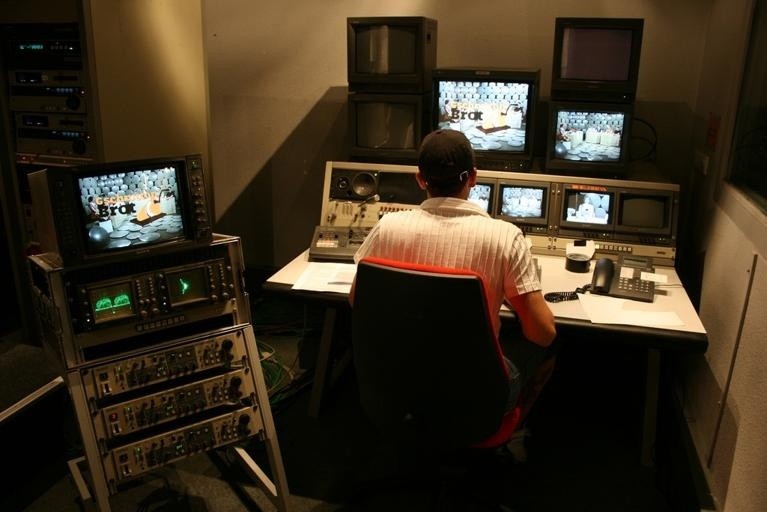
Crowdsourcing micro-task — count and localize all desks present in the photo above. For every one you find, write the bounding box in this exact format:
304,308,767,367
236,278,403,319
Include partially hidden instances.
261,247,708,467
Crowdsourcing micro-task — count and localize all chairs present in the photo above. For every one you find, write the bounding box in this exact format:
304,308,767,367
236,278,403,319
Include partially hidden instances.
352,259,533,511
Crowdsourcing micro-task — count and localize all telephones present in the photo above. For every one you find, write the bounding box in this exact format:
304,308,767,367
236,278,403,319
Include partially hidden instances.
590,251,655,303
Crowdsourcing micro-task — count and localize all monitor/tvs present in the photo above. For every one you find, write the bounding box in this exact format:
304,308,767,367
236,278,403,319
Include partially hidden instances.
467,181,494,217
346,17,438,89
551,16,644,100
346,90,425,164
558,183,614,242
496,178,552,235
47,153,214,271
615,188,679,249
544,97,635,180
430,69,541,173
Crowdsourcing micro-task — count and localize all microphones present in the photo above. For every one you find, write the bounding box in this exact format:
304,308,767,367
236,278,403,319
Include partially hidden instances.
357,194,380,207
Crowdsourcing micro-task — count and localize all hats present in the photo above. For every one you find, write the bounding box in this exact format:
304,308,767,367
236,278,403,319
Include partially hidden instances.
417,129,475,185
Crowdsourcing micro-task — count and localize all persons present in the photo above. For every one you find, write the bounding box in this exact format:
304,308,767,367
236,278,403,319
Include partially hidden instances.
514,102,523,112
578,196,594,217
88,196,97,215
444,99,452,120
559,124,565,136
348,129,558,464
614,127,621,134
